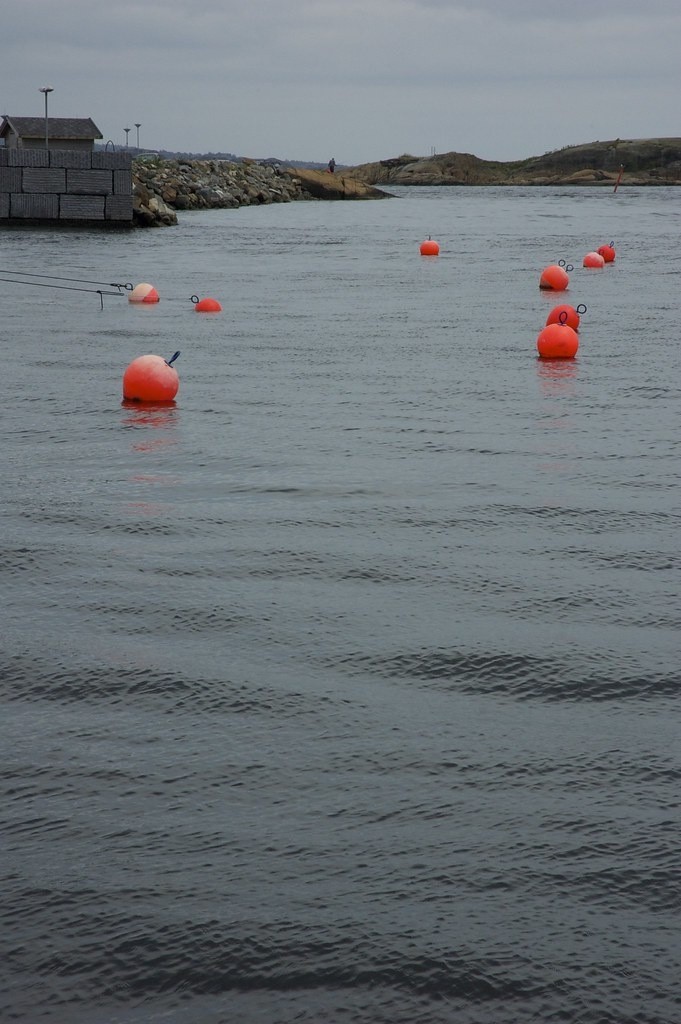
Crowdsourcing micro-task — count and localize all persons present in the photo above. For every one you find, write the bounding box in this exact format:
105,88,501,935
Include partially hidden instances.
327,158,336,172
